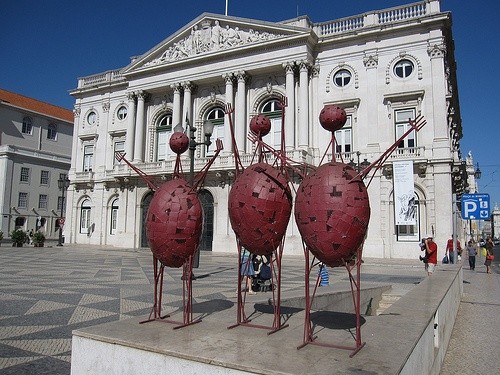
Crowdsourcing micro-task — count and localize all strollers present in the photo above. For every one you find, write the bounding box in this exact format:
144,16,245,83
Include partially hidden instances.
250,253,277,292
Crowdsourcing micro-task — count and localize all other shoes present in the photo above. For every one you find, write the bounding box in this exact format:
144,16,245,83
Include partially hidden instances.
249,290,257,295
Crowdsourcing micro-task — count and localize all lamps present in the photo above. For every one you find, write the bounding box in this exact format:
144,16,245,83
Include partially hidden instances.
473,162,482,179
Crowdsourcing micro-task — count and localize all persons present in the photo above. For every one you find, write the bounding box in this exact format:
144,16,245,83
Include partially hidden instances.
421,234,495,275
235,246,271,295
24,229,34,245
317,261,330,287
158,21,268,63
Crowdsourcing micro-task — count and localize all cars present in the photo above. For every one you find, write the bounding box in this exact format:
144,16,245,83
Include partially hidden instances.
494,239,500,245
479,238,486,247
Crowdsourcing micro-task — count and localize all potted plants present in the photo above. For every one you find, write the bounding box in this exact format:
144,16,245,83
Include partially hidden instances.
31,230,46,247
10,229,28,247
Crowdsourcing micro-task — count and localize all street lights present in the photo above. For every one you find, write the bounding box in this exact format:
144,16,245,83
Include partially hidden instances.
173,119,215,281
347,151,371,263
56,176,71,247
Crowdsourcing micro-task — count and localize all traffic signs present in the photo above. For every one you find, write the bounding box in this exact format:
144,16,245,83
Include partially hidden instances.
460,193,490,220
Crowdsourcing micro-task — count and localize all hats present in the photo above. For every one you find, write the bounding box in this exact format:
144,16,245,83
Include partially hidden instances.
426,235,433,239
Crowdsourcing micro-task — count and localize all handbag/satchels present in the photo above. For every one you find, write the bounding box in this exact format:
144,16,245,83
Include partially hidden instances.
443,257,448,263
486,255,495,260
457,254,461,261
